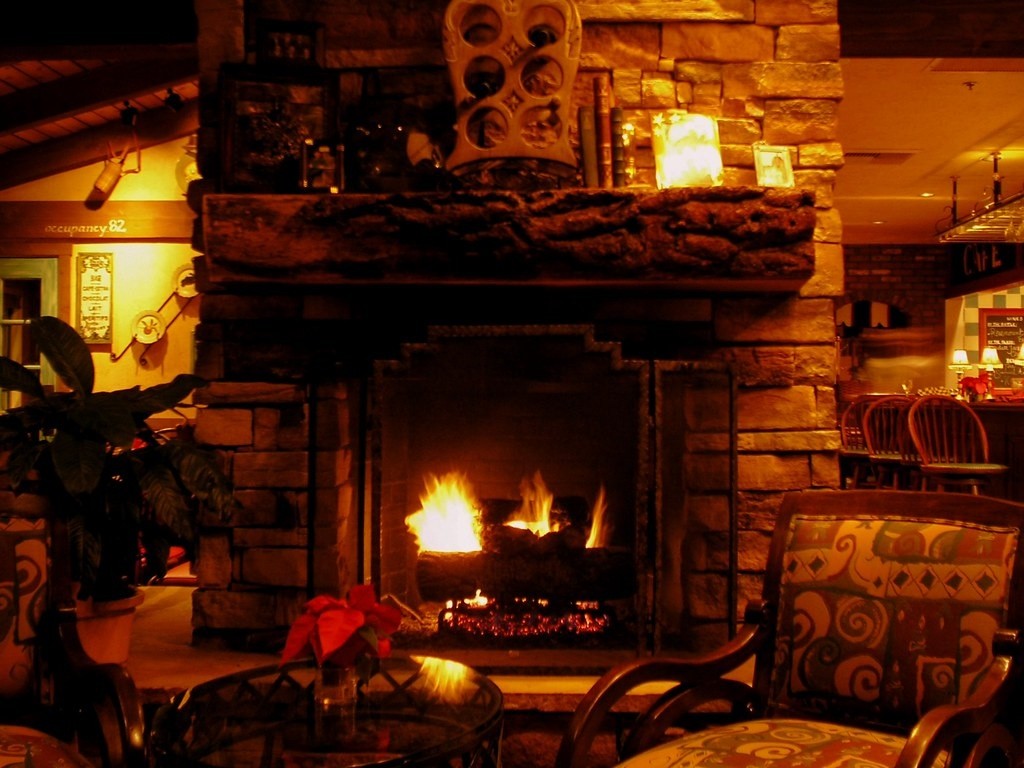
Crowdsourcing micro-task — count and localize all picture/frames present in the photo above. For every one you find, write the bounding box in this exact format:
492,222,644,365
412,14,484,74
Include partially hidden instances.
754,144,796,189
75,253,114,343
300,136,344,193
976,306,1024,400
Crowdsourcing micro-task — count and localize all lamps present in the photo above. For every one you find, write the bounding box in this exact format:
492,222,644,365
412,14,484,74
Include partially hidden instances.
936,153,1023,244
83,140,141,211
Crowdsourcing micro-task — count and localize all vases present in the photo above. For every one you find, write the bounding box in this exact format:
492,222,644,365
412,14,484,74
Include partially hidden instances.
58,585,137,664
311,661,358,720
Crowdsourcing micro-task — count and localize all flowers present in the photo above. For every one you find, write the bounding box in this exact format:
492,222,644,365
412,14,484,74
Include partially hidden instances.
0,316,295,589
286,585,399,666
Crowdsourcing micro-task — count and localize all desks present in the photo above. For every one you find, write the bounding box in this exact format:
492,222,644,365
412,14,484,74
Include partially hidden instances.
144,655,505,768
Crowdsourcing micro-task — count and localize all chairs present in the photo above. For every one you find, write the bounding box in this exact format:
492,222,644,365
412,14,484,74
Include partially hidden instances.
863,394,918,488
842,406,874,488
907,395,1007,495
556,499,1024,768
0,494,142,768
